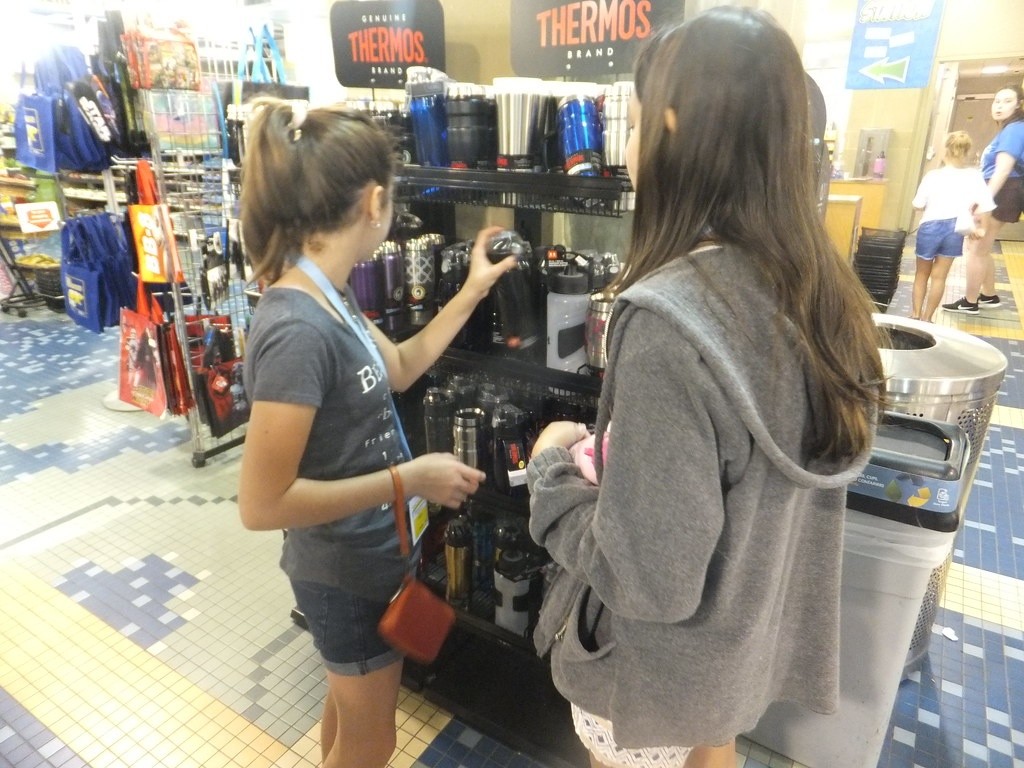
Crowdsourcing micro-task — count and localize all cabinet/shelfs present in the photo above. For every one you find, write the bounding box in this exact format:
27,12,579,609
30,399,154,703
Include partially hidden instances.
375,163,631,668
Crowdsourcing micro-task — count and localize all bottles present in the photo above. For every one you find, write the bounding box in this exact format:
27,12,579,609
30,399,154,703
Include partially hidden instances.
476,382,509,406
451,376,477,402
554,400,582,422
424,387,454,453
492,403,528,499
438,247,464,316
444,503,531,645
341,65,493,202
517,382,555,435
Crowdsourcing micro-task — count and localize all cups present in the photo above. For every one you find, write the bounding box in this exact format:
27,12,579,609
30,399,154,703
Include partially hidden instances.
456,227,621,396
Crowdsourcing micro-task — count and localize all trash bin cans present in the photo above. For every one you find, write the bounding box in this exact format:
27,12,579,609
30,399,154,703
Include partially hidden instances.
870,314,1010,688
735,409,970,768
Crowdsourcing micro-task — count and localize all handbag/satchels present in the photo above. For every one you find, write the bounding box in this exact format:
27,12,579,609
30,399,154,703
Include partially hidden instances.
377,465,455,664
952,208,984,241
14,37,252,441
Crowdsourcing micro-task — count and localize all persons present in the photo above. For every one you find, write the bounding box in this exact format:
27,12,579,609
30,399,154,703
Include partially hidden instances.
942,85,1024,315
909,130,997,323
526,6,884,768
241,101,516,768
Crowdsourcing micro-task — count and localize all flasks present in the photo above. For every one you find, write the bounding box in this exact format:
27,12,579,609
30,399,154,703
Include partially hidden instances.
493,77,637,205
348,233,444,333
453,407,488,470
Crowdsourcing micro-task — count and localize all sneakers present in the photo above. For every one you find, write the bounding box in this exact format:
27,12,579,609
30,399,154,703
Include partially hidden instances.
942,297,981,316
977,292,1000,310
